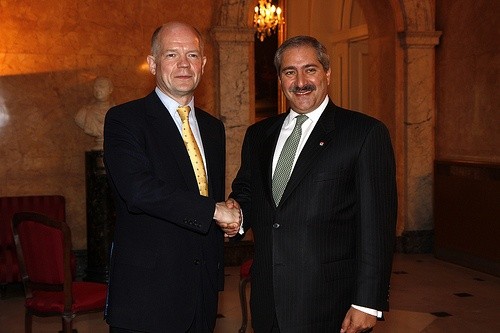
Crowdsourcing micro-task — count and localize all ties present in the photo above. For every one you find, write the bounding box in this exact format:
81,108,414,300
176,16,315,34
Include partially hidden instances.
271,115,309,207
176,106,209,198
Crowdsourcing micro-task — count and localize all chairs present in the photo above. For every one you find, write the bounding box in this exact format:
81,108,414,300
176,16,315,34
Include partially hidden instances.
238,258,254,333
11,210,107,333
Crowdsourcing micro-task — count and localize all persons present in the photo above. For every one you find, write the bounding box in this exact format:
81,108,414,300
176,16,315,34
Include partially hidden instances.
75,75,120,138
102,21,240,332
218,35,398,333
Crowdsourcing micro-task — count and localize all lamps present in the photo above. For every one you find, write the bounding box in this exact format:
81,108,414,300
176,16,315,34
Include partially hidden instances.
254,0,284,41
